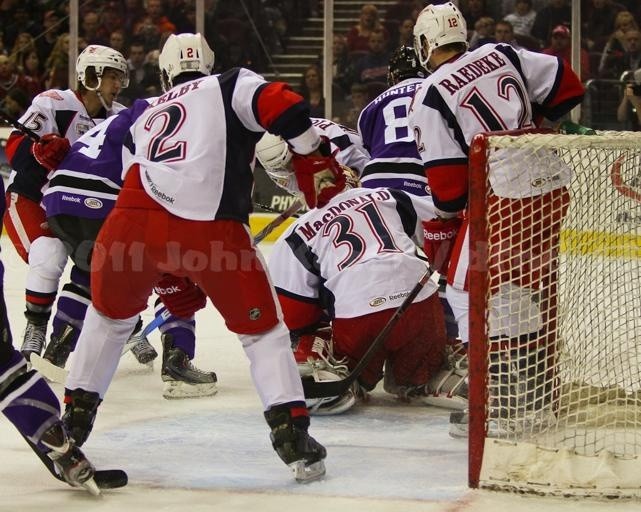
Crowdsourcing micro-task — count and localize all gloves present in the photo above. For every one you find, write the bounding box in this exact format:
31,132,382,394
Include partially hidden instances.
289,135,346,208
153,274,207,319
29,134,70,171
421,215,463,276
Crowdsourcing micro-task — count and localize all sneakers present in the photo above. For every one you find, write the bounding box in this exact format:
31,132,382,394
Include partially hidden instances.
39,388,103,487
21,312,217,383
264,407,326,467
304,350,555,424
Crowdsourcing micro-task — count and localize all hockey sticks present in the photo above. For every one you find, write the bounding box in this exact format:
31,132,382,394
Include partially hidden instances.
302,268,437,399
30,176,332,388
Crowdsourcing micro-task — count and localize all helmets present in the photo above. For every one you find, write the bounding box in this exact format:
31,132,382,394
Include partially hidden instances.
74,44,130,91
386,1,471,89
158,32,215,94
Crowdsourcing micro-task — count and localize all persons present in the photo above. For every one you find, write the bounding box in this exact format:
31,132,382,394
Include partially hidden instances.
0,1,641,489
